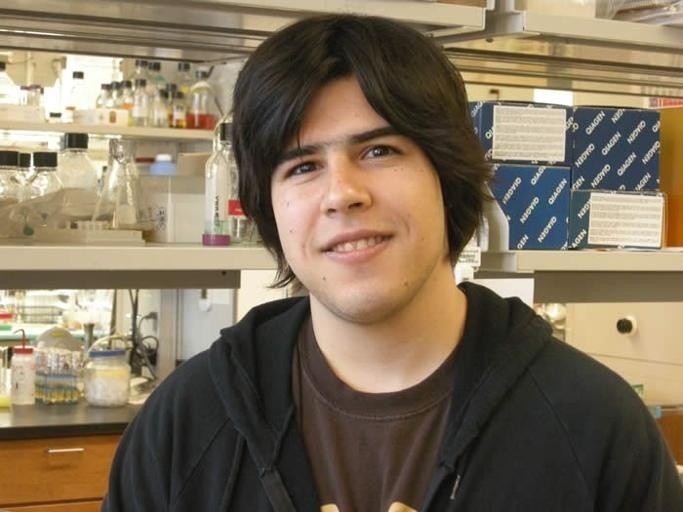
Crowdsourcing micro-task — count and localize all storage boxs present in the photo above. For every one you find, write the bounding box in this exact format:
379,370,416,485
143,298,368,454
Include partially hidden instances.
468,97,682,252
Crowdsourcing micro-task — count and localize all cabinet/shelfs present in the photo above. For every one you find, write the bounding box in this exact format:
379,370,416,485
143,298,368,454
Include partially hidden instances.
525,272,681,405
1,117,290,292
0,427,122,510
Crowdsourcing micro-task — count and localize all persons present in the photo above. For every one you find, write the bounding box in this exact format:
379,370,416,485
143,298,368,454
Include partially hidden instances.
101,14,682,512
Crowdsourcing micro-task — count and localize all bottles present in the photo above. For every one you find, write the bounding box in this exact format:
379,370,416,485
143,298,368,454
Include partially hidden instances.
0,39,246,248
0,291,133,408
